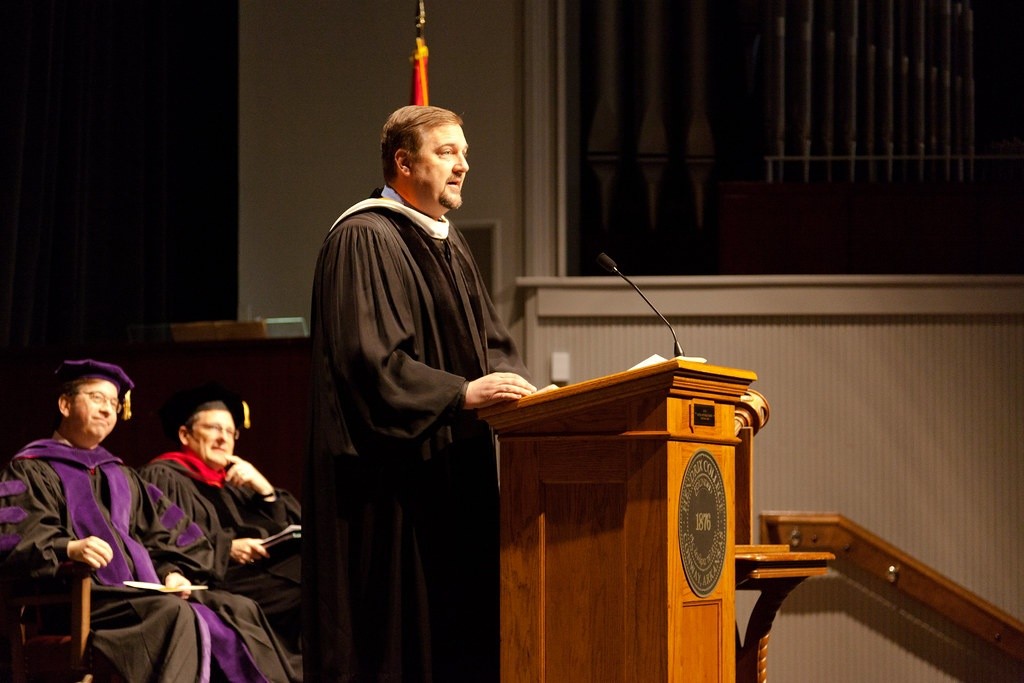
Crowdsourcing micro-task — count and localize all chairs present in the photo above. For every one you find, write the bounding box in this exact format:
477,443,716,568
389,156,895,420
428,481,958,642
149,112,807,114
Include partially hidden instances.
10,576,122,683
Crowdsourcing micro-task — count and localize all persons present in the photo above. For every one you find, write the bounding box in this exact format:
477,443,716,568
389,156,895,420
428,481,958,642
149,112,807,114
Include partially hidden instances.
310,106,537,683
0,360,303,683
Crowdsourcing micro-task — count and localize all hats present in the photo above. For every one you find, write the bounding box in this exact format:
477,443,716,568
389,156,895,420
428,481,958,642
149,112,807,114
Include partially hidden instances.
52,360,134,420
157,382,251,444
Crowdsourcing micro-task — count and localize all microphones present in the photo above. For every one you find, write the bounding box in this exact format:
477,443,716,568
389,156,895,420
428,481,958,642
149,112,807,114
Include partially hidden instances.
599,253,685,357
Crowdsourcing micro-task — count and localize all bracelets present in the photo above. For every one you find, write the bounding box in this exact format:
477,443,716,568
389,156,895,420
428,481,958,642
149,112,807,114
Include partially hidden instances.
264,493,274,498
